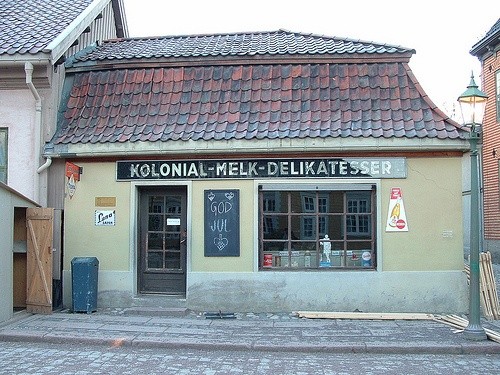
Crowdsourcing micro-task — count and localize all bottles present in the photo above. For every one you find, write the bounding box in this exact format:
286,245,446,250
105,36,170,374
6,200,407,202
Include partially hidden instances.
304,250,311,270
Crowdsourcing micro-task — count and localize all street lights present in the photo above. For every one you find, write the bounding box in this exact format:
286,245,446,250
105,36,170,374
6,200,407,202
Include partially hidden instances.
456,71,488,342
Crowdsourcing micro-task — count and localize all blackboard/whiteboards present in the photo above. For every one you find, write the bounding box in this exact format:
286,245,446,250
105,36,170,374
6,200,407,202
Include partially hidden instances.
204,189,241,257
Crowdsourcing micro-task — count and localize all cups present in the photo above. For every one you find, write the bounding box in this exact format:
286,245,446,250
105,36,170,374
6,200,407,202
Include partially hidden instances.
275,256,281,267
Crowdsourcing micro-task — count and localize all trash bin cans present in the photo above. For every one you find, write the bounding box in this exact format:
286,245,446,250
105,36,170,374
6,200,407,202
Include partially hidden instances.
70,257,99,314
